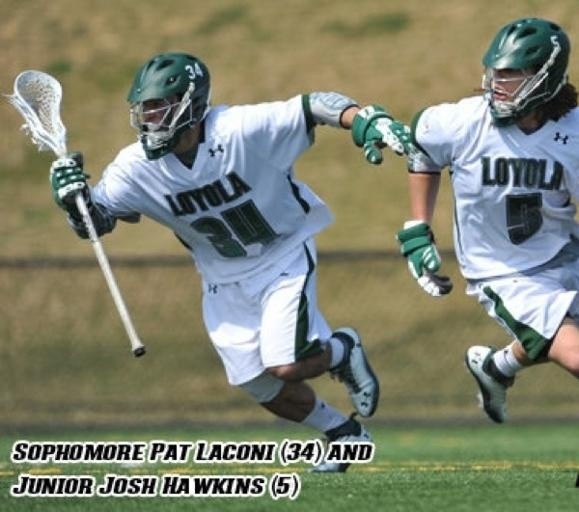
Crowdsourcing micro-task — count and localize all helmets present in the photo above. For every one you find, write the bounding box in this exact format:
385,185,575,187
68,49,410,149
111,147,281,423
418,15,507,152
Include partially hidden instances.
480,17,571,128
127,52,211,161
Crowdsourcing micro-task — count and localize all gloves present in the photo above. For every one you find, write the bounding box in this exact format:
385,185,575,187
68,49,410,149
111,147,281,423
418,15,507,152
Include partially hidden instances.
395,222,454,299
48,152,96,221
350,104,416,166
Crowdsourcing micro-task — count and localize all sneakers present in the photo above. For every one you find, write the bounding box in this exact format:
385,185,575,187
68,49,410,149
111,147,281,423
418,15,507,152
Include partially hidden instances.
465,343,516,424
330,326,380,418
308,413,374,473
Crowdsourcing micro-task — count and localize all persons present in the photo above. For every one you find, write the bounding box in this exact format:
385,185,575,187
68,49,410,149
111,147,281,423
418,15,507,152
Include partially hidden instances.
394,15,579,428
47,49,419,474
